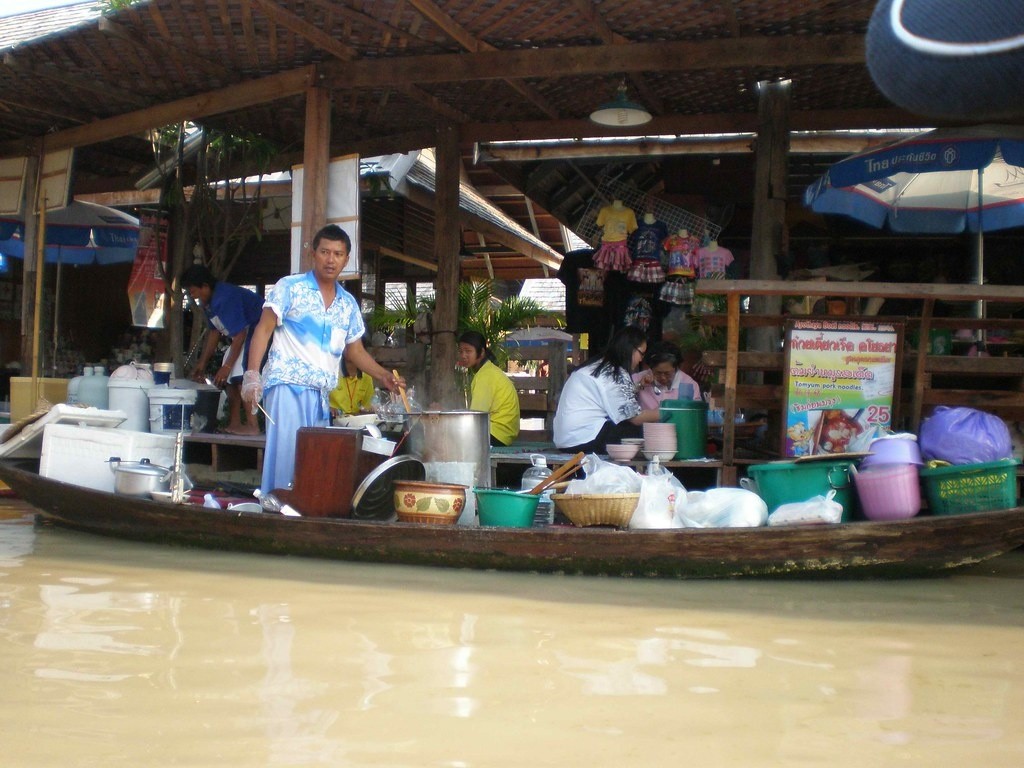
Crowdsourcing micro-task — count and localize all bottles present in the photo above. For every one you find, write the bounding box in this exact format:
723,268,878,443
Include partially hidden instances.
520,454,556,524
709,407,747,439
203,494,222,509
252,489,303,516
67,366,109,410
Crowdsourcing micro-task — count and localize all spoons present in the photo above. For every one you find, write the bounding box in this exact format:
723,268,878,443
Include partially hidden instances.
650,380,662,395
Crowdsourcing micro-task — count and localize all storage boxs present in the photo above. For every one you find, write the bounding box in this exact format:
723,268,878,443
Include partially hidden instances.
0,403,182,493
783,315,897,463
915,458,1021,517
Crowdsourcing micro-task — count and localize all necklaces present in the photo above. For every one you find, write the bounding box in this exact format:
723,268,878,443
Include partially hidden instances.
345,373,358,410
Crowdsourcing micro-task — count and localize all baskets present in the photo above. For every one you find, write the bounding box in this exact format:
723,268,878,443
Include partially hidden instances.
549,490,639,529
854,465,921,521
920,457,1021,518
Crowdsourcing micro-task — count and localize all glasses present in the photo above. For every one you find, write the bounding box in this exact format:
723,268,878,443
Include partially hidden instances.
633,345,646,360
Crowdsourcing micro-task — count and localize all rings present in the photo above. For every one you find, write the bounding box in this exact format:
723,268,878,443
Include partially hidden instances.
222,378,224,383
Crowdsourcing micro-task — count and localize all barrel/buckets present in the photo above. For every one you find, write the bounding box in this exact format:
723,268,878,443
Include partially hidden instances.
746,461,857,524
847,464,922,522
106,381,154,430
658,400,708,460
154,363,176,384
145,389,197,434
174,387,222,434
472,485,542,529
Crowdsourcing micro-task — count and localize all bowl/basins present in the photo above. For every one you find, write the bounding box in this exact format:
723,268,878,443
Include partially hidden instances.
150,491,190,504
606,422,678,462
333,414,396,432
227,503,263,513
393,479,470,525
859,438,925,470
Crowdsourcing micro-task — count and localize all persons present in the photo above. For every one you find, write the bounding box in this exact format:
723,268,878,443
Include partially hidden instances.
552,326,659,455
430,331,521,448
695,239,735,298
178,264,273,436
241,223,407,510
660,228,701,306
329,359,379,425
590,198,639,272
632,342,701,417
626,213,669,283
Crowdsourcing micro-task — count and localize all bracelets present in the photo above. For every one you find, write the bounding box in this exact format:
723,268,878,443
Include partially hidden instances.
224,363,232,371
639,382,647,393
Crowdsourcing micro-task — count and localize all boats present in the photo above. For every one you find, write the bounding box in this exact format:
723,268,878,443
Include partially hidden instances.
0,456,1022,577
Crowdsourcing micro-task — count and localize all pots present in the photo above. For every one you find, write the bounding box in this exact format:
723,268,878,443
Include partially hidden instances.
109,456,176,499
400,411,496,489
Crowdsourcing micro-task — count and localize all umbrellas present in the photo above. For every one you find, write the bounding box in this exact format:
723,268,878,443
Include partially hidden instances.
0,201,143,375
803,123,1024,340
503,325,580,361
